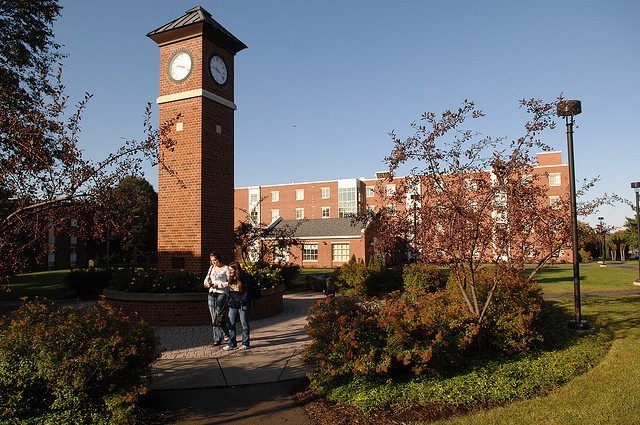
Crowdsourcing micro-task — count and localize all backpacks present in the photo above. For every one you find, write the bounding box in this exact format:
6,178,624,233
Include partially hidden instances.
244,272,263,309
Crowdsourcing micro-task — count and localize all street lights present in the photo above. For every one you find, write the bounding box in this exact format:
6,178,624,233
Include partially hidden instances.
556,98,591,330
598,216,607,267
630,181,640,285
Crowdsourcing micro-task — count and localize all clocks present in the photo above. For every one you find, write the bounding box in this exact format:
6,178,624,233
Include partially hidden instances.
167,52,192,82
210,56,228,85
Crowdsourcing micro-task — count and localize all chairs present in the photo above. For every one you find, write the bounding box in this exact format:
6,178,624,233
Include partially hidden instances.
48,263,55,270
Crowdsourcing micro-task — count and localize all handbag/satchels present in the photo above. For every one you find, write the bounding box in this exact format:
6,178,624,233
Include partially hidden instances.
212,301,229,327
204,264,214,288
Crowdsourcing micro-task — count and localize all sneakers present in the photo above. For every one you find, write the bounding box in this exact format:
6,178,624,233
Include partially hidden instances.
239,344,250,350
222,345,236,351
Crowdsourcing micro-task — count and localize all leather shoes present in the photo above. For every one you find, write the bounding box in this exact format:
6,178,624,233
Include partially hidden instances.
223,337,229,342
211,341,222,347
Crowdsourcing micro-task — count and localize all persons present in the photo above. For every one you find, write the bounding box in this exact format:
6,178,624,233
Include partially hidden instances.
203,251,230,347
223,262,253,350
